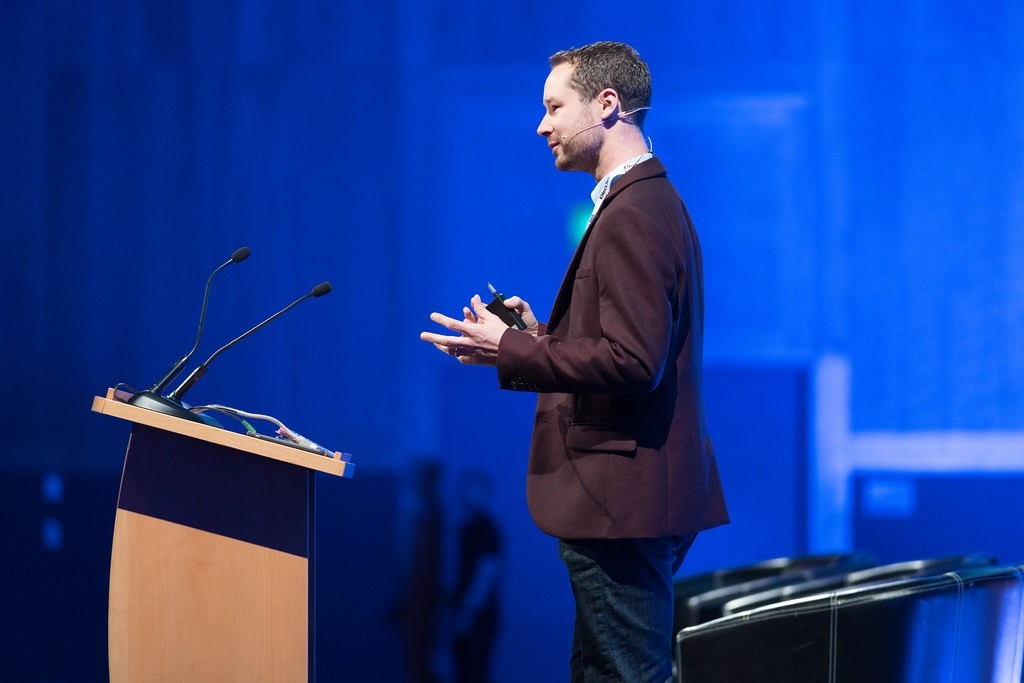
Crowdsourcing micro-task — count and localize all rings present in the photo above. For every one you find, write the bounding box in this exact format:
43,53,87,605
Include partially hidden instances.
453,346,464,357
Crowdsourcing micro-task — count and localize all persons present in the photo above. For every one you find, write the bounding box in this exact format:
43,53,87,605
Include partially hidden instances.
418,39,731,683
385,456,504,683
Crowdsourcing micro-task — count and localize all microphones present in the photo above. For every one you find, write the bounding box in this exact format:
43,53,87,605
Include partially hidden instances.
166,282,333,428
562,116,620,139
127,246,251,425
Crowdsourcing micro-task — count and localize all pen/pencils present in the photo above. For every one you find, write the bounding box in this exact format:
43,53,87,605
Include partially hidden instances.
487,282,524,331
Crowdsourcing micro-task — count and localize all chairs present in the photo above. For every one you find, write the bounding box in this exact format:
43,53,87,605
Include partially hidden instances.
673,547,859,683
675,561,1024,683
686,553,999,625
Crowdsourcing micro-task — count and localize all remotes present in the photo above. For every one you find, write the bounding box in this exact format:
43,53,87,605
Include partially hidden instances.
485,283,528,331
